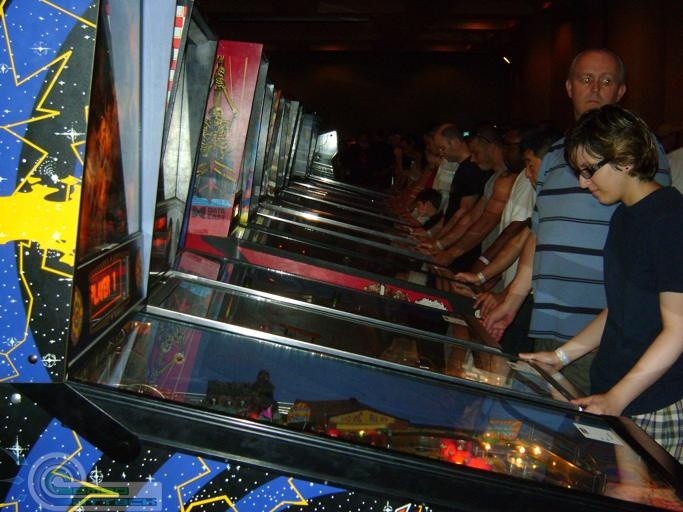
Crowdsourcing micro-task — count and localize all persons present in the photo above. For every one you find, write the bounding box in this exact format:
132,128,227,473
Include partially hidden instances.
336,122,563,357
456,358,569,482
602,426,651,505
515,104,682,470
481,46,677,400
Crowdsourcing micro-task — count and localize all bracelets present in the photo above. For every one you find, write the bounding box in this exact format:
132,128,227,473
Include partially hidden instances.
552,347,571,368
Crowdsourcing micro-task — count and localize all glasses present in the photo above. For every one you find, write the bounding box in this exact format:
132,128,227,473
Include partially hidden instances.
580,157,610,179
